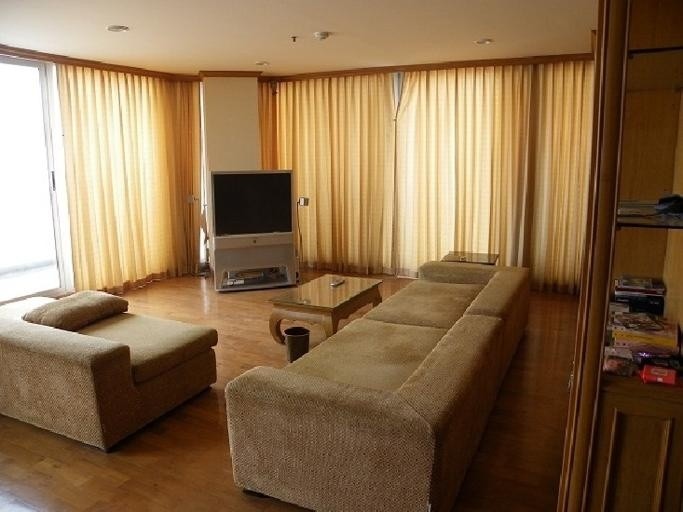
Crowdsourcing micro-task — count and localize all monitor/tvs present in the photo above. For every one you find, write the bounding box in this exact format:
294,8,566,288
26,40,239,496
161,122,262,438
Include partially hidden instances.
207,169,298,249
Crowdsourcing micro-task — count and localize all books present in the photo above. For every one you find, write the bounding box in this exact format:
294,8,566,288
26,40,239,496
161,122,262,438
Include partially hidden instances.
617,203,656,216
602,275,679,385
617,213,665,225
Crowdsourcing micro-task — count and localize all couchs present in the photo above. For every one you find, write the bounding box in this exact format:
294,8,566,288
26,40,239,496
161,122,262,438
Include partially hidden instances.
225,261,530,512
0,288,218,453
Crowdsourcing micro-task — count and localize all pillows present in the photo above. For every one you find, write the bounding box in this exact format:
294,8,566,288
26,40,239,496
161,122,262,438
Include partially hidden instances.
21,290,128,331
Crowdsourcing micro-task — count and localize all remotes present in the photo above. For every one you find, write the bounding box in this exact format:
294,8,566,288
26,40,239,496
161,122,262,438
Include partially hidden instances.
329,278,345,286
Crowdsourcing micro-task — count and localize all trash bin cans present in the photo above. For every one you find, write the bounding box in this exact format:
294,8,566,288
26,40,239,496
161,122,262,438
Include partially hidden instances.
283,326,310,363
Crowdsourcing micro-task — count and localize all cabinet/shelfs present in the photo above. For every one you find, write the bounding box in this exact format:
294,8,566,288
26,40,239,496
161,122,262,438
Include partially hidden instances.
556,0,683,512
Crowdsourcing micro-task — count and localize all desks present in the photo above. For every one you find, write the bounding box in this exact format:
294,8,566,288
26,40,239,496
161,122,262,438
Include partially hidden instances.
440,251,500,265
